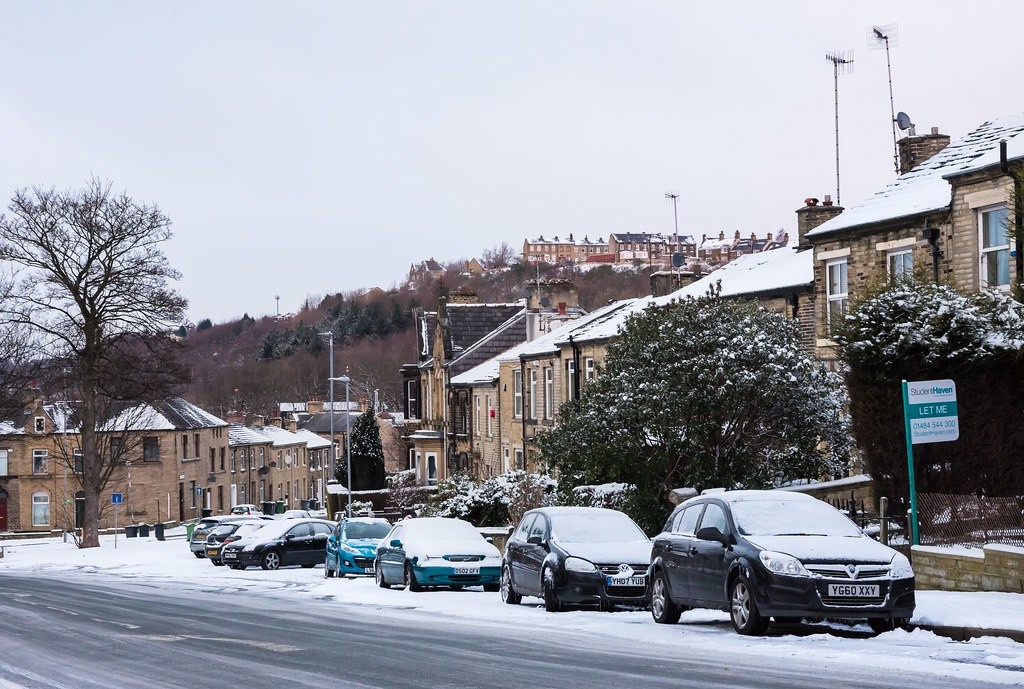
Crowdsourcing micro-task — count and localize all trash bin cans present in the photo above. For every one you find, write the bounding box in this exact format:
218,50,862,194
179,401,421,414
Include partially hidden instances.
154,524,164,541
261,502,276,515
139,525,149,537
301,499,310,510
276,501,284,513
201,509,212,518
309,498,317,511
186,524,195,541
124,526,138,538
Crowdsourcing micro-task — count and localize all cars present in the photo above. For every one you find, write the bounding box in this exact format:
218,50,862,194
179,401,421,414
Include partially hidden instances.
499,506,656,613
648,490,916,636
373,517,503,592
220,519,338,570
325,517,394,578
190,503,328,567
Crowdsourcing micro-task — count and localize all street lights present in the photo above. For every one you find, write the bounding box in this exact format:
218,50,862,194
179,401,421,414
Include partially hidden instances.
317,330,335,481
327,374,354,518
